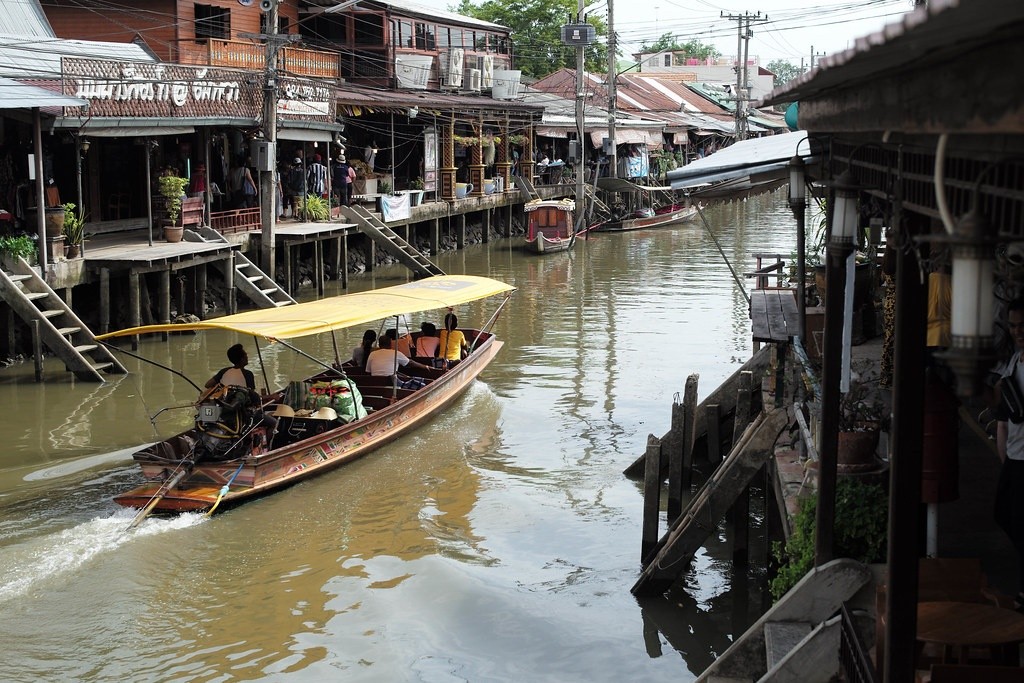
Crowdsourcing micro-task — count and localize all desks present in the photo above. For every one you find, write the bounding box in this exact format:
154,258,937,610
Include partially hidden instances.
351,179,378,202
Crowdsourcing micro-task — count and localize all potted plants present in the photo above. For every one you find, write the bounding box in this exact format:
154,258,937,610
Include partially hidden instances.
158,175,190,244
64,205,95,259
296,192,330,223
835,356,890,466
0,232,38,268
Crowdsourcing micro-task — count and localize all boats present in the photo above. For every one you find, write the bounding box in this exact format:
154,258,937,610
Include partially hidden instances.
583,176,705,234
93,273,521,535
521,196,577,256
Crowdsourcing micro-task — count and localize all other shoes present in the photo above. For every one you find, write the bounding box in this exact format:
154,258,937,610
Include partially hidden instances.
279,213,287,221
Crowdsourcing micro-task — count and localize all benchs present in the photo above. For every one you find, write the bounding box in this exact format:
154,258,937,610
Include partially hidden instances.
351,190,422,213
310,365,449,414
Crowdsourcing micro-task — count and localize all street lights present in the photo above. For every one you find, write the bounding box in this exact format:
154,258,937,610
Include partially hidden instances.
259,0,364,285
609,46,677,202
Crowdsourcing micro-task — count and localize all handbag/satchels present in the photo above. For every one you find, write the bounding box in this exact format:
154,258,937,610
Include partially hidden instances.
245,179,254,194
1001,376,1024,424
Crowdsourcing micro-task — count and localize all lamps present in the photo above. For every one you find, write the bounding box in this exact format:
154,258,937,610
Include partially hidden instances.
80,138,90,157
788,136,825,221
947,154,1024,395
826,143,896,267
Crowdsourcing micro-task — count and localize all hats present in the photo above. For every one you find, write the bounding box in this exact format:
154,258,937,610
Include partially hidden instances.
193,163,206,170
293,158,303,163
312,154,321,161
335,155,347,163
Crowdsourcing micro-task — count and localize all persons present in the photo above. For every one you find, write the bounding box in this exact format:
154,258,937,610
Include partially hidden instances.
191,165,205,228
232,159,256,206
998,298,1024,613
205,343,278,441
509,143,553,176
275,149,356,222
417,314,468,362
666,139,720,159
352,329,430,389
365,142,375,171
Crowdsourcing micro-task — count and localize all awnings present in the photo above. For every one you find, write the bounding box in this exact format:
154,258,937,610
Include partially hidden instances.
667,129,810,303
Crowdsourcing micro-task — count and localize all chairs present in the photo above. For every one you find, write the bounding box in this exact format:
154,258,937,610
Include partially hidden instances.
108,192,132,220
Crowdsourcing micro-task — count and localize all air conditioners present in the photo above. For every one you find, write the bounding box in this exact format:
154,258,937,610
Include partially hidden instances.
448,48,494,92
